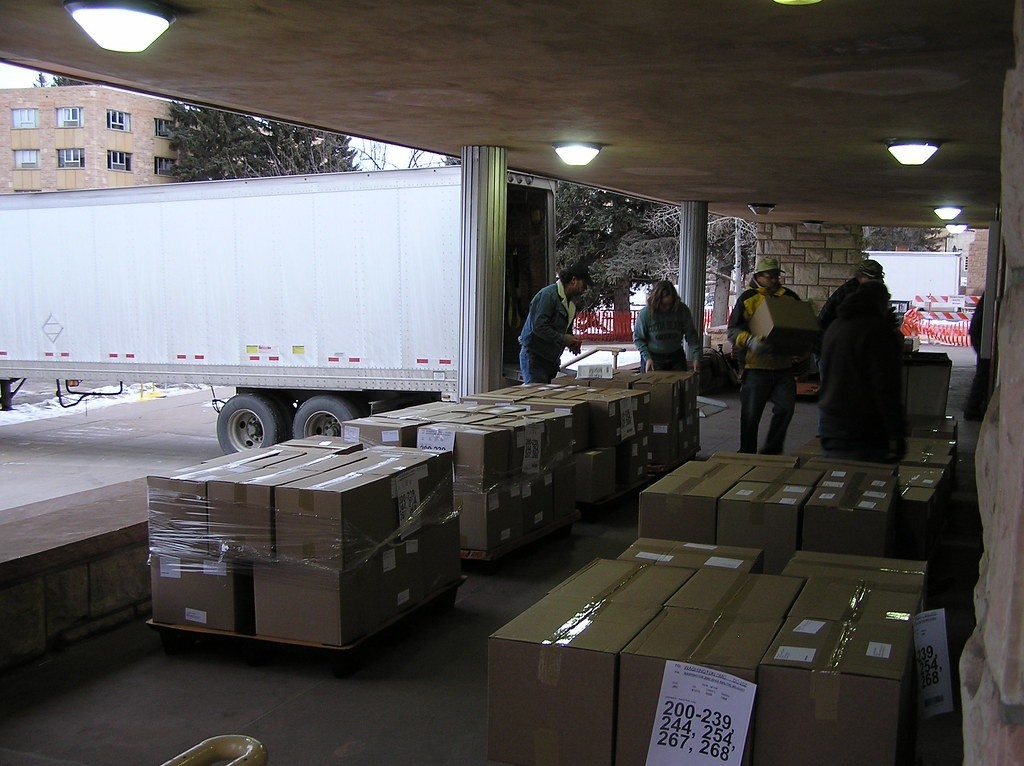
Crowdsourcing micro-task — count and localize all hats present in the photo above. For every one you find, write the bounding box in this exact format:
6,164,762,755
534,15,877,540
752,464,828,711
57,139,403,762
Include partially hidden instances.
571,263,595,287
755,257,786,273
859,260,885,285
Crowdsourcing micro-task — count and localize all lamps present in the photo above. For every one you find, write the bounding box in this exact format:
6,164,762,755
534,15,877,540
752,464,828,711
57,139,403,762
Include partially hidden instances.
551,142,602,166
882,137,943,166
748,203,776,215
63,0,177,53
933,206,976,245
802,220,824,231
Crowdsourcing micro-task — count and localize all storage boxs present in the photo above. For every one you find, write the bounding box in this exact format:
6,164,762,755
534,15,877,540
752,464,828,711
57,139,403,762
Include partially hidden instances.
143,298,960,766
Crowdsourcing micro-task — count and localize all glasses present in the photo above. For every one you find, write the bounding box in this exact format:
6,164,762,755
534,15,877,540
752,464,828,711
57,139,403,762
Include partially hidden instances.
762,275,782,280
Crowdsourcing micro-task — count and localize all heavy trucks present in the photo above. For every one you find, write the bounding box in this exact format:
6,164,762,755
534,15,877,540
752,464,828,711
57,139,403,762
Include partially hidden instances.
0,166,562,456
705,251,961,320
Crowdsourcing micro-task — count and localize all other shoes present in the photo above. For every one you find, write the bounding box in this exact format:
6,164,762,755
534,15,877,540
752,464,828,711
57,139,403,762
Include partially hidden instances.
963,413,984,421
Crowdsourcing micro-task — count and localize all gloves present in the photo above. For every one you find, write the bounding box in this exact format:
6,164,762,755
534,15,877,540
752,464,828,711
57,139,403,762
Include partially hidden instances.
745,335,774,354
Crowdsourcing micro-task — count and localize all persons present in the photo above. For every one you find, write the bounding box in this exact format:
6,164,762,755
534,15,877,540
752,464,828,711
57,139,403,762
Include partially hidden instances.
632,281,703,373
519,265,595,385
962,292,991,419
813,260,909,463
726,258,802,455
